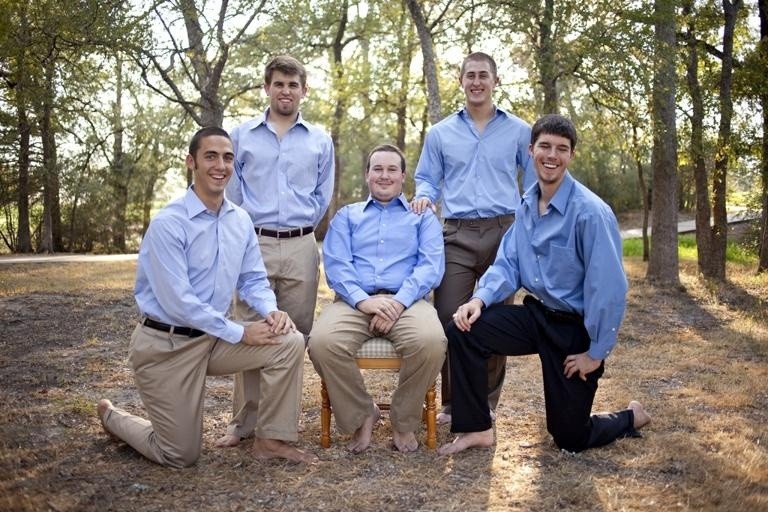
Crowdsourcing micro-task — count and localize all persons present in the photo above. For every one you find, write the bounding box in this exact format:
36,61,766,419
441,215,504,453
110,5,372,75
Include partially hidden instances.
307,144,445,452
98,130,318,467
409,53,540,425
437,115,650,453
217,58,334,446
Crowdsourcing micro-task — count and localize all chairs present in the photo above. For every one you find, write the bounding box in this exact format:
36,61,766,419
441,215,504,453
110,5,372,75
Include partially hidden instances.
321,339,438,451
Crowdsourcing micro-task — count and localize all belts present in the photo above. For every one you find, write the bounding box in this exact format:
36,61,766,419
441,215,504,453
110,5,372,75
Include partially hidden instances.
254,225,313,239
364,288,398,295
445,214,514,226
144,318,205,337
